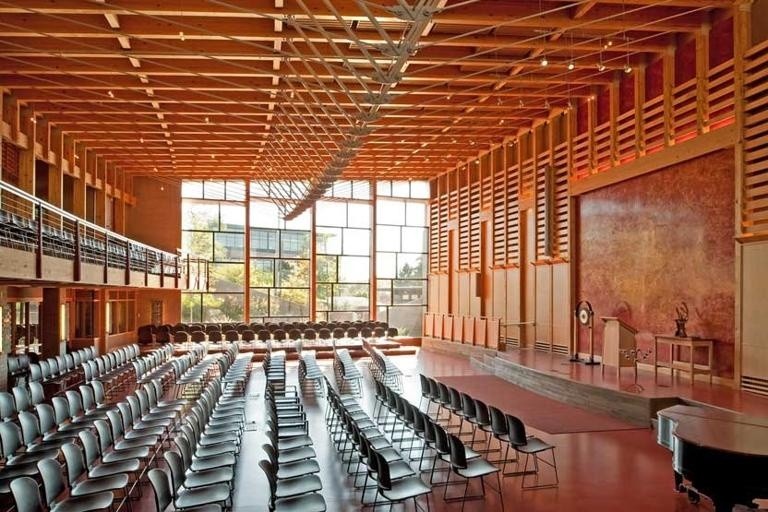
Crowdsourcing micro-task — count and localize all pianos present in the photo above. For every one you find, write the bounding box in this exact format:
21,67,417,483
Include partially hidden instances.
655,404,768,511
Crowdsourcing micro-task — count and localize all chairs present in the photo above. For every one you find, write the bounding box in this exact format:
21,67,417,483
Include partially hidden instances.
1,210,177,275
131,318,401,339
0,345,562,510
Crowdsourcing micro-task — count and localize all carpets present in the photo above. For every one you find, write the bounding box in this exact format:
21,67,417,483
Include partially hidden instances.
434,370,649,435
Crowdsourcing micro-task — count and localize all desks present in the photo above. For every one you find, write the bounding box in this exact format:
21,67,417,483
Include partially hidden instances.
651,334,714,389
